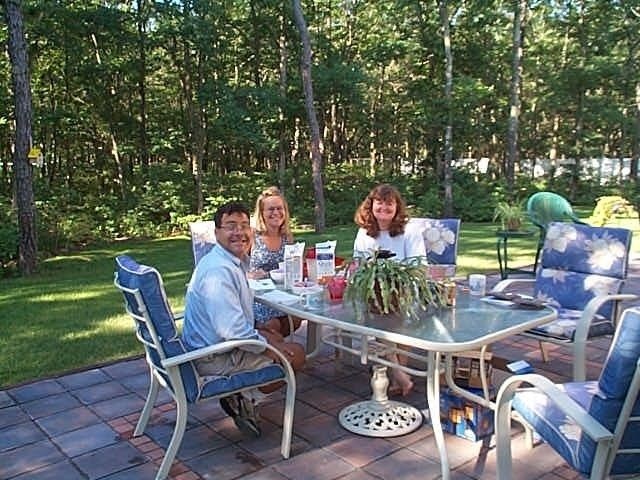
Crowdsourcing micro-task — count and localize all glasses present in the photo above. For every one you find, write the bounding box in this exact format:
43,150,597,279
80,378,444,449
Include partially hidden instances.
218,222,251,234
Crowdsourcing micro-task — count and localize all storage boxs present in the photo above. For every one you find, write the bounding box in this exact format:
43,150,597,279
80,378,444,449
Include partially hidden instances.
428,350,498,442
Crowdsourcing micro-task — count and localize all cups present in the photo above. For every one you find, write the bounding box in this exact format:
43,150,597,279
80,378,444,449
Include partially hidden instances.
469,274,487,297
299,288,324,311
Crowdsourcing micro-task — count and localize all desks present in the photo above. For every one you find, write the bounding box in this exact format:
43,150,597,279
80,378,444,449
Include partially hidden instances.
253,280,558,439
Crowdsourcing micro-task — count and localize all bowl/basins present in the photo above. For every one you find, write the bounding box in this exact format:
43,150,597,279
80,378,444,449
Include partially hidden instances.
291,281,318,294
270,269,284,283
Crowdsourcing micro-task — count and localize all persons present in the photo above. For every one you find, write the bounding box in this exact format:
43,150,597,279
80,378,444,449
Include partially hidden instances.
351,184,428,398
181,186,306,438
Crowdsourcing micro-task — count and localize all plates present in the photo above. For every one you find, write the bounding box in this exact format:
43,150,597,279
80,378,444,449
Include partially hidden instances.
479,292,532,307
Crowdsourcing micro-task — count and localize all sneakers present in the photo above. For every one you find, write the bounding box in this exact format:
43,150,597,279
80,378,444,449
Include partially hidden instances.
219,392,261,434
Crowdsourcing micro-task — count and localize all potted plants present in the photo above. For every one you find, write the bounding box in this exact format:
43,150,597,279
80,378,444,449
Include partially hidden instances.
321,245,455,323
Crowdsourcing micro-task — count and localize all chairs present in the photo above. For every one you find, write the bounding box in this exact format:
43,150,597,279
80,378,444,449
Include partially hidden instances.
114,254,297,479
491,307,640,479
341,216,461,366
188,217,295,346
492,220,640,382
493,192,592,280
